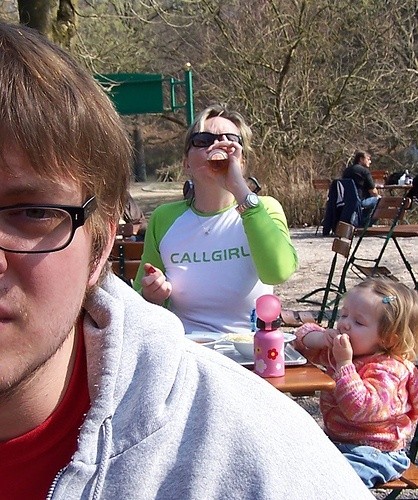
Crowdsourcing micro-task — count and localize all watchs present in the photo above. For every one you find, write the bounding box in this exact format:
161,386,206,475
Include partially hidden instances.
235,192,259,214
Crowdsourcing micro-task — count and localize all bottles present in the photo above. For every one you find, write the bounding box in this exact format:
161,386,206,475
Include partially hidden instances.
254,294,285,378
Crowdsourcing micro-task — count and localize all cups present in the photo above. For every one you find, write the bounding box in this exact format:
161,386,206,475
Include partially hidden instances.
207,148,229,170
398,180,404,185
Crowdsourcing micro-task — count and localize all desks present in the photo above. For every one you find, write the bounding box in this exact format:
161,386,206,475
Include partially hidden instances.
246,362,335,397
355,225,417,290
375,185,413,196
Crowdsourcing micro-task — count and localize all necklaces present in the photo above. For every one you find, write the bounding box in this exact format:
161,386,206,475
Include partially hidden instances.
196,210,226,235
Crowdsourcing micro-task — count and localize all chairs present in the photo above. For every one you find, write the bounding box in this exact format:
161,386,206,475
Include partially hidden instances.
374,420,418,500
279,170,417,328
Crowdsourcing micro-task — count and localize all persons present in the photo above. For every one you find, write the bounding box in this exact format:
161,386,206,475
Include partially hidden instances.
344,150,382,207
293,272,418,489
0,20,376,500
133,103,299,334
113,192,145,243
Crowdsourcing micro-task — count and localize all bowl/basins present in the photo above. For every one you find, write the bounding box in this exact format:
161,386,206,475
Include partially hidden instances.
226,332,297,359
185,334,224,349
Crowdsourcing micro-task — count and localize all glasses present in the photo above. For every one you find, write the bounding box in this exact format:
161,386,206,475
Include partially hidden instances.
0,195,99,254
185,132,244,155
